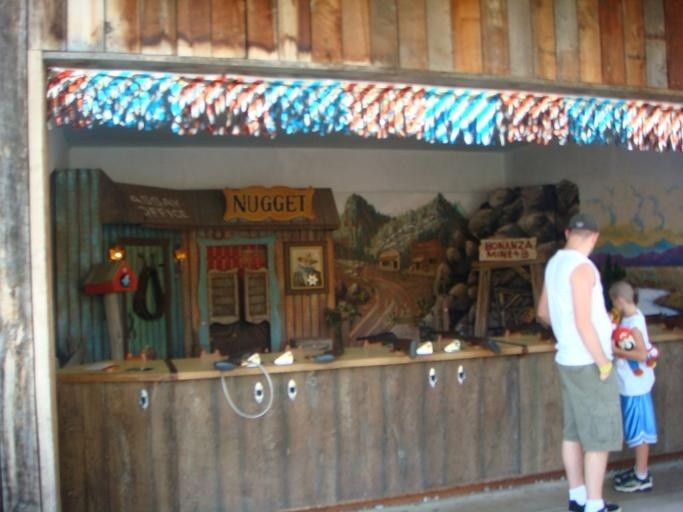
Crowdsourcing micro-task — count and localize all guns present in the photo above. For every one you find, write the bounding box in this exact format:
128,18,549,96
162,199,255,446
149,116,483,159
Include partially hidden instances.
509,316,553,341
355,332,416,359
644,314,682,330
424,328,502,354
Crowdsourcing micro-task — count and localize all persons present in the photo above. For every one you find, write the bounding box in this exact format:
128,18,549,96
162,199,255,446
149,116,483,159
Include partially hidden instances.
609,280,657,493
612,328,658,376
538,214,623,512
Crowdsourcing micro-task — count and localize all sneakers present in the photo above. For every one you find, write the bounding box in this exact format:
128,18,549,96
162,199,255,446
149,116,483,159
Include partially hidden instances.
568,499,621,512
614,467,654,482
613,479,654,493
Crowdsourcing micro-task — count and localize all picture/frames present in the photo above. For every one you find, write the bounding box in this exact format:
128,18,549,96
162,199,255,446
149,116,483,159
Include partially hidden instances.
280,238,328,296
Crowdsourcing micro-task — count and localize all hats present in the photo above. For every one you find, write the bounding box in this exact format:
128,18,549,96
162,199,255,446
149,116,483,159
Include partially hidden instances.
567,213,597,232
